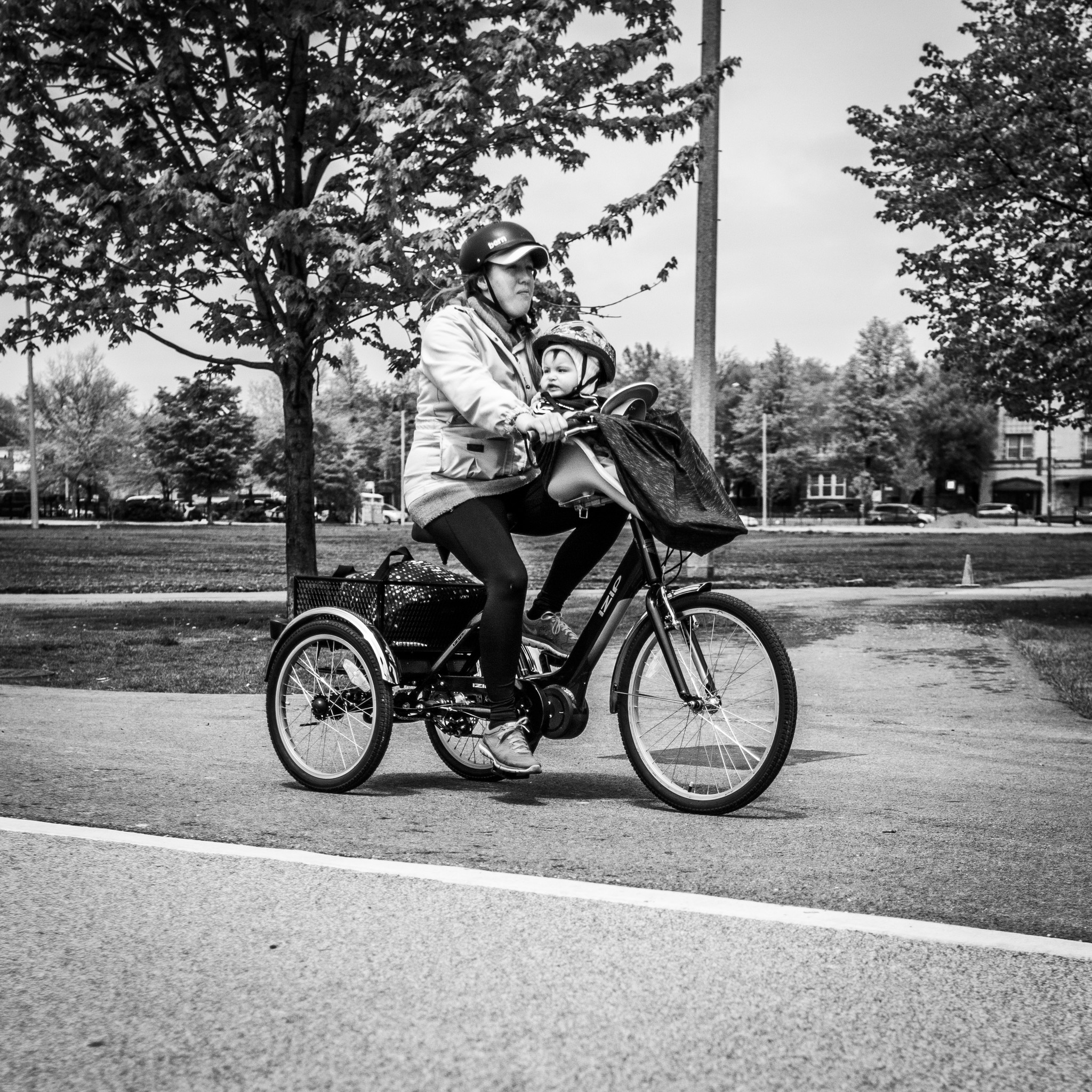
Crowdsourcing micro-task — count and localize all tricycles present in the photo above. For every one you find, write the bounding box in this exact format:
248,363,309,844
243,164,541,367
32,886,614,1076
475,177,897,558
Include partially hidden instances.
262,405,799,815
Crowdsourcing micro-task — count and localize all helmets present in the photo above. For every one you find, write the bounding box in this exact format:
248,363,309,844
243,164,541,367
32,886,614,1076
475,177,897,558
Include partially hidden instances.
531,321,616,388
459,222,543,299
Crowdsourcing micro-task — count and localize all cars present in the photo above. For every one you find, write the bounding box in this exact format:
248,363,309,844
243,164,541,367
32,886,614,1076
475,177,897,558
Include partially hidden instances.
1034,506,1092,527
977,503,1024,519
803,502,847,519
382,503,409,525
868,504,935,527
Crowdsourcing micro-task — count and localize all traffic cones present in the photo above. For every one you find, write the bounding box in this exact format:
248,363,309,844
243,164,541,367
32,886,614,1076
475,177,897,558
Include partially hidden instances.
955,553,981,588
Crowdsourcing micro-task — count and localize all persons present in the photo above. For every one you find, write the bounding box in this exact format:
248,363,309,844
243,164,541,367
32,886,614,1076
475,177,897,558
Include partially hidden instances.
403,223,631,775
526,319,644,520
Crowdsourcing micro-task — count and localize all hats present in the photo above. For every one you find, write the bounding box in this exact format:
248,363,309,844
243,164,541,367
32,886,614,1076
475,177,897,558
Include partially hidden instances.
483,244,550,270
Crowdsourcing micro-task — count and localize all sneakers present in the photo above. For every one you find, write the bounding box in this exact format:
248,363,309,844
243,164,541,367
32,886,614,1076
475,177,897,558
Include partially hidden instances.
522,610,578,658
478,721,543,774
